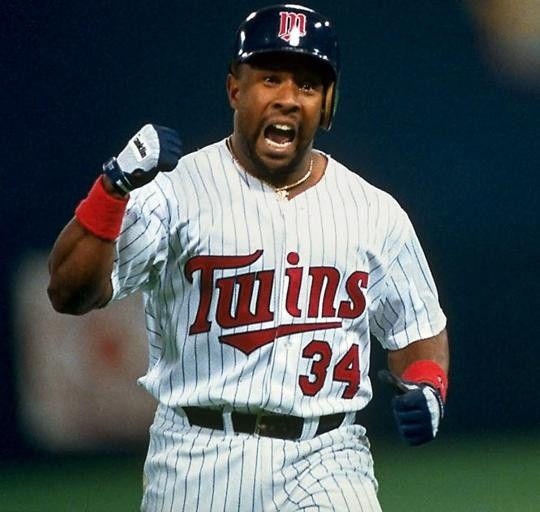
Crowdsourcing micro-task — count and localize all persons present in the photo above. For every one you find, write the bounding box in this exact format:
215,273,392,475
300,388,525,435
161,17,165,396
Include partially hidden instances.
47,2,449,512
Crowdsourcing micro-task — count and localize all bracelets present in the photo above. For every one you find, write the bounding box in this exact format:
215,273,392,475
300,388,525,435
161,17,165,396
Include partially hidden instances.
75,175,130,241
400,359,448,404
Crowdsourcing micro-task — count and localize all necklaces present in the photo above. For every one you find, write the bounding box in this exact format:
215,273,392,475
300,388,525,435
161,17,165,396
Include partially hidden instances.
227,134,314,201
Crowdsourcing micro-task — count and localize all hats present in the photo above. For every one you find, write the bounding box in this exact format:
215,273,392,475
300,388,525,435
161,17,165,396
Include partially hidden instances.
231,4,338,81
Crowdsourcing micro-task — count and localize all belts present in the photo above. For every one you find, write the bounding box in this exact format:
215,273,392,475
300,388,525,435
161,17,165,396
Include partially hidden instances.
184,404,345,439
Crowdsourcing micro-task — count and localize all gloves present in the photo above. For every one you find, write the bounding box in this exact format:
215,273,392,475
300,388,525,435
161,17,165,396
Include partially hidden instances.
102,120,185,197
377,368,445,447
